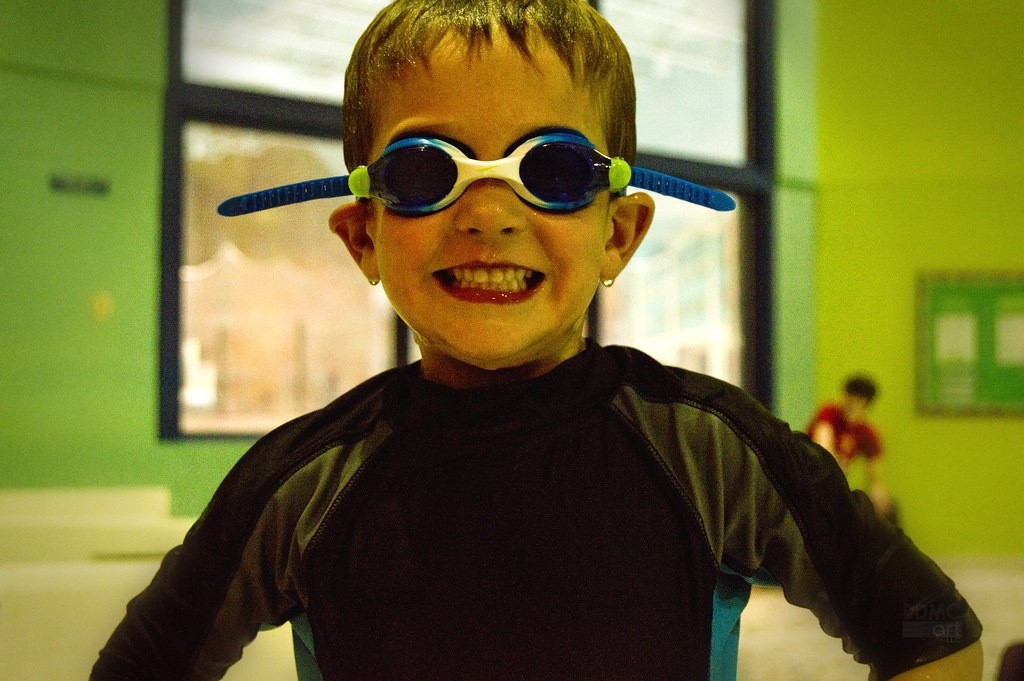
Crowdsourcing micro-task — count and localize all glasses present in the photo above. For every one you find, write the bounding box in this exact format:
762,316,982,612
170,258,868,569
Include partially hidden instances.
219,126,736,218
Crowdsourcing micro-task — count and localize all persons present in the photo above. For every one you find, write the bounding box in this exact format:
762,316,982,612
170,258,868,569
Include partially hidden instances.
87,1,985,681
808,374,903,517
